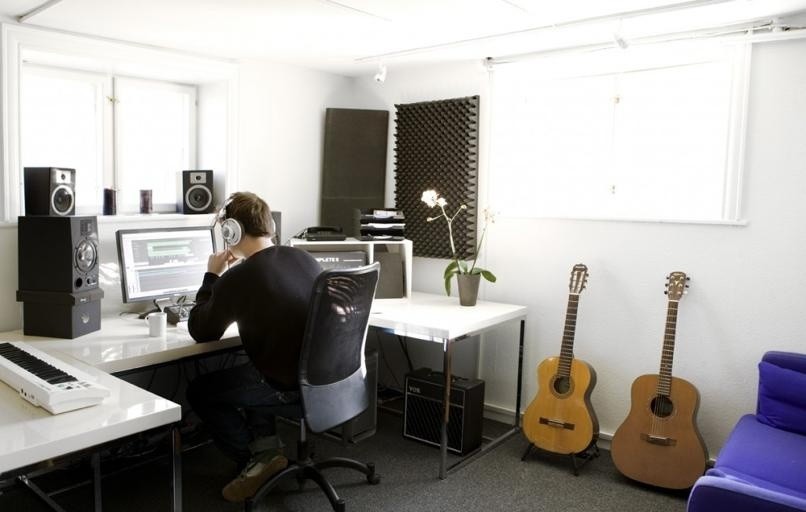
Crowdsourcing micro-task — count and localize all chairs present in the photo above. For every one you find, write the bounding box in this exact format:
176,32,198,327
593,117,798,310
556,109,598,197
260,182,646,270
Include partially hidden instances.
236,262,386,512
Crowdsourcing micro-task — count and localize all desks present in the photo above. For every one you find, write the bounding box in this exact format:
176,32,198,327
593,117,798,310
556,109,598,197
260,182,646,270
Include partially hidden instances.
0,291,530,511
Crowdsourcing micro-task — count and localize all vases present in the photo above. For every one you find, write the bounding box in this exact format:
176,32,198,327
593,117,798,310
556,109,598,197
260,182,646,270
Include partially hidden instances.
456,270,480,306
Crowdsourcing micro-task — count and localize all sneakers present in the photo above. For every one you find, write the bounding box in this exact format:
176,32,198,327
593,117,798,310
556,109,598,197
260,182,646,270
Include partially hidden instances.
222,450,288,502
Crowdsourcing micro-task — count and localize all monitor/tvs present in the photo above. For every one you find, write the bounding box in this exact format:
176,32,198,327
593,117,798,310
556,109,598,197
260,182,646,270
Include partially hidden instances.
115,226,216,319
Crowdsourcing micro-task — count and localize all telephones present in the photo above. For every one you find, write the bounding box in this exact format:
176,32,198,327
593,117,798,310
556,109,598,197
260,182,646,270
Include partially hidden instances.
305,226,347,241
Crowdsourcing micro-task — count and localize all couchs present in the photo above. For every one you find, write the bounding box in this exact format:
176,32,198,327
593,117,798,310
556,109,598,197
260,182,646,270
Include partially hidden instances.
686,351,806,512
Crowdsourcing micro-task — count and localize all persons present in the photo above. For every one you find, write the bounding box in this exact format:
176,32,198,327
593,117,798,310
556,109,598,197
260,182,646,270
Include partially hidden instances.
187,192,325,503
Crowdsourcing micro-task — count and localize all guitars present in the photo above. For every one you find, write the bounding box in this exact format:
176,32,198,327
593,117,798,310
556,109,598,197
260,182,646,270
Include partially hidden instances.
521,263,600,455
611,271,710,489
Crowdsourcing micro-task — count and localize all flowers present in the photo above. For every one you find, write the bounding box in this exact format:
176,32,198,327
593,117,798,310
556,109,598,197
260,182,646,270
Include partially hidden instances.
420,189,498,298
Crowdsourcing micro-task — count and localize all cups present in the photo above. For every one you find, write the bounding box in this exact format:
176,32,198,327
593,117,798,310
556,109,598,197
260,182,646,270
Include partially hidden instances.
145,312,168,339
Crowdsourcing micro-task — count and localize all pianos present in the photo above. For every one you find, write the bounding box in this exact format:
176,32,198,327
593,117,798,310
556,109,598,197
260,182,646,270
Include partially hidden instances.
0,340,104,415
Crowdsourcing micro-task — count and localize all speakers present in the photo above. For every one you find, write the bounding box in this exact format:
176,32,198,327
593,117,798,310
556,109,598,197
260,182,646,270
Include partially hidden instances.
402,368,484,454
182,169,213,215
24,166,76,216
18,215,99,291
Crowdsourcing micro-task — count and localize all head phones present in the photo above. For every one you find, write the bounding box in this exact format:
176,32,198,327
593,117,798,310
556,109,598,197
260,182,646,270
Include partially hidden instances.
218,201,276,246
